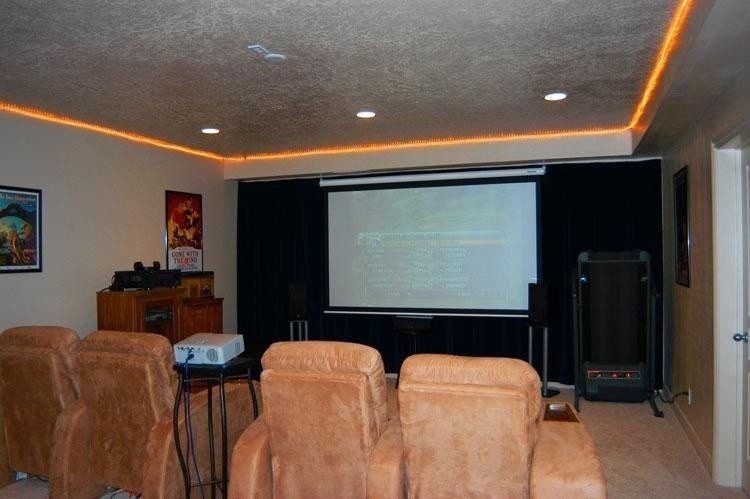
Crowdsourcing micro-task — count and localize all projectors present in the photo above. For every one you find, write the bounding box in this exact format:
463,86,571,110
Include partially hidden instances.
174,332,246,366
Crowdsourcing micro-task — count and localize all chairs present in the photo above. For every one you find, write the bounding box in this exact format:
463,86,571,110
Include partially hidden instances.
52,329,265,499
1,323,81,499
365,350,607,497
226,339,405,498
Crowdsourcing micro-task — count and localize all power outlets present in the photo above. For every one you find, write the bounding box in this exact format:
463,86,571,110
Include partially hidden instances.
686,386,692,406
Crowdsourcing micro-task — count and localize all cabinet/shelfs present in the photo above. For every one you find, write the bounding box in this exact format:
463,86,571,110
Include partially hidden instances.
96,285,186,342
183,295,225,339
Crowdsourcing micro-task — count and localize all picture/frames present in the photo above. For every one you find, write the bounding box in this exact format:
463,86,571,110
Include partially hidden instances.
164,189,205,273
1,183,44,274
672,162,691,289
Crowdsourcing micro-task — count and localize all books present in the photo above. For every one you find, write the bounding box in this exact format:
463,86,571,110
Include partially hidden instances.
146,308,173,322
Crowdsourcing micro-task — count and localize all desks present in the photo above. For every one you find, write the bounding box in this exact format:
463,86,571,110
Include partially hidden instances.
171,357,260,499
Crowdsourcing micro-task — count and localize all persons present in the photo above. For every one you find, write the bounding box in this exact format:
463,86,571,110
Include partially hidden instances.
191,281,209,295
168,196,201,247
0,222,37,265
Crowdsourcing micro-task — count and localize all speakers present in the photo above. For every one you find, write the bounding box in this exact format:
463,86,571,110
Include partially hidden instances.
582,360,647,403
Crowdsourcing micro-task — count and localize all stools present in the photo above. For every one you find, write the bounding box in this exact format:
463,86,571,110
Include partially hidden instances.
288,314,309,343
